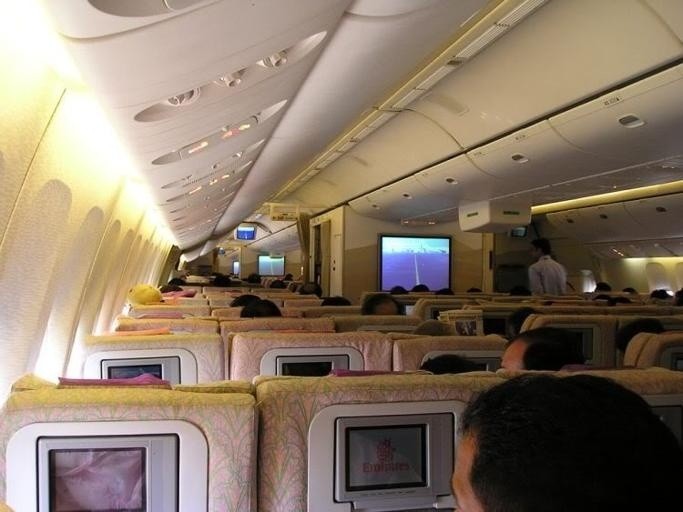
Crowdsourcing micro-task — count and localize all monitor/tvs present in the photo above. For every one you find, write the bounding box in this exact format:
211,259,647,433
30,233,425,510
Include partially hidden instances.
233,261,239,275
237,226,255,240
378,233,452,292
457,320,476,336
37,434,178,512
276,355,348,377
468,357,502,371
566,327,595,359
257,254,285,277
335,414,453,510
101,357,180,386
483,318,506,335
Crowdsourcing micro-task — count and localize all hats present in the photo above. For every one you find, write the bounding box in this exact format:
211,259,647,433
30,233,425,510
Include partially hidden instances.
126,283,165,307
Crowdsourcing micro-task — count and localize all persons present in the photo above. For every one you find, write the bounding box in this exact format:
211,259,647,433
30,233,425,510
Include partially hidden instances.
526,236,566,297
152,267,681,378
448,371,681,510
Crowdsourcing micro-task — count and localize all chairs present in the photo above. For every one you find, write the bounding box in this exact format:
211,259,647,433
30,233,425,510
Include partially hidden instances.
251,370,510,510
82,278,682,388
512,367,682,451
1,377,264,510
